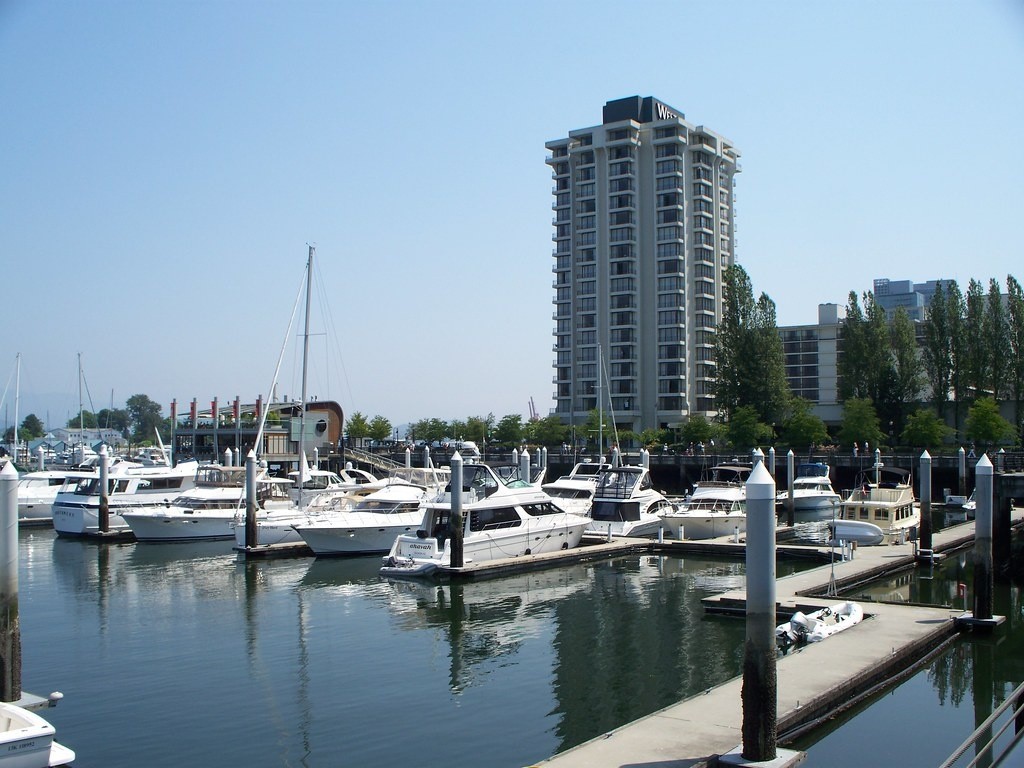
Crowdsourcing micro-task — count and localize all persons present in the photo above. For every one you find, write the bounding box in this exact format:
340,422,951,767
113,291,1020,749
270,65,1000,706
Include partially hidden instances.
478,437,999,463
178,420,265,456
364,440,452,456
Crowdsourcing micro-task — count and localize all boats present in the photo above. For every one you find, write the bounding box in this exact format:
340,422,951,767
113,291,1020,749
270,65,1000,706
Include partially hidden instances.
0,701,75,768
0,244,924,581
774,603,864,647
962,486,976,519
